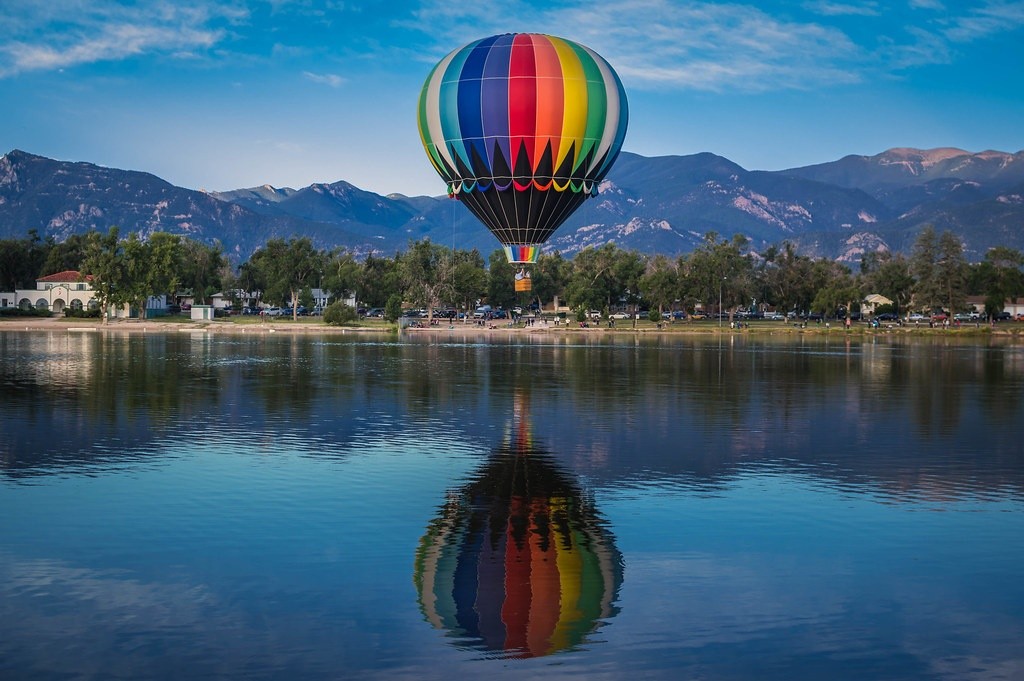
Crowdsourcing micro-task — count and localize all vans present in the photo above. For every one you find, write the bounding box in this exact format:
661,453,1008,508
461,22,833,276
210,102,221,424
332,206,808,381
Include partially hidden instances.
584,310,602,319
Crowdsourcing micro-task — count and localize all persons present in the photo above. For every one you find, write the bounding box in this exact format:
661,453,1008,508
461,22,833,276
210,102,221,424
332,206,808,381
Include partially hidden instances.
942,318,949,329
929,319,937,329
794,322,802,328
977,320,979,328
897,317,902,327
673,315,675,323
593,316,599,326
816,319,820,328
843,318,846,329
449,315,453,328
992,319,995,328
486,311,492,319
868,316,881,329
514,315,521,324
579,321,589,328
956,318,961,330
730,321,749,329
411,319,439,328
608,316,615,328
529,316,570,326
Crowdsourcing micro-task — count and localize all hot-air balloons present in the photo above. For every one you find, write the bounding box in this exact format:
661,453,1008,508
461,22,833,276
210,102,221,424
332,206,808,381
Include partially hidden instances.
409,388,628,659
413,32,630,292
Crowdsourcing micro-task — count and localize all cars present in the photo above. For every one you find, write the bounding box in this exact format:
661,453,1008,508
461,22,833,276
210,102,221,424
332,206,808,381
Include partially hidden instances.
842,312,863,320
639,311,649,318
715,308,788,322
929,313,948,320
691,312,707,320
182,303,507,320
609,312,630,319
903,313,923,322
954,310,1024,322
673,311,687,319
875,313,898,321
802,313,820,320
662,311,670,319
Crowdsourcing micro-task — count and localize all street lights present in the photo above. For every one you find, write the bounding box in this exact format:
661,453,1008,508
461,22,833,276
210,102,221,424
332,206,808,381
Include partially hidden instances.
719,276,727,328
